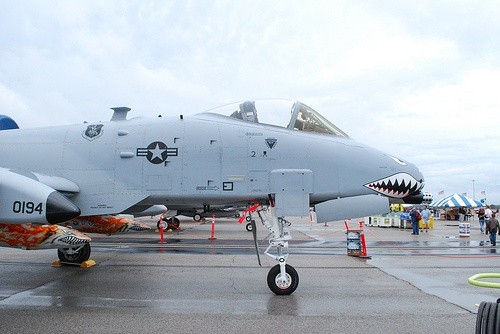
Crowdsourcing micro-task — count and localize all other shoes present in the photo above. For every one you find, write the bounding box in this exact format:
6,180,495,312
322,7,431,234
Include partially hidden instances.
412,233,416,234
417,233,418,234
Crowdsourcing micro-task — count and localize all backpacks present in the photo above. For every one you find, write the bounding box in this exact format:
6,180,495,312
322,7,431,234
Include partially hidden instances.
415,212,421,220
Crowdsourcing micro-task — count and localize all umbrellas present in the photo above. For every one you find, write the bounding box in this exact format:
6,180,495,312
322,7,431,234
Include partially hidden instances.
429,193,486,221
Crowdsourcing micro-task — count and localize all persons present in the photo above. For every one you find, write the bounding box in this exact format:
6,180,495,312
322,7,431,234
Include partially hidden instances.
478,208,487,234
486,214,500,246
420,205,431,232
463,209,472,221
409,208,419,235
436,207,440,217
433,207,437,217
459,207,467,215
480,205,493,235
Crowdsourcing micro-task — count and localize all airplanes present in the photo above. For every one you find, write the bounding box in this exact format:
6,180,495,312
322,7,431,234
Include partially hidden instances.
0,97,434,295
